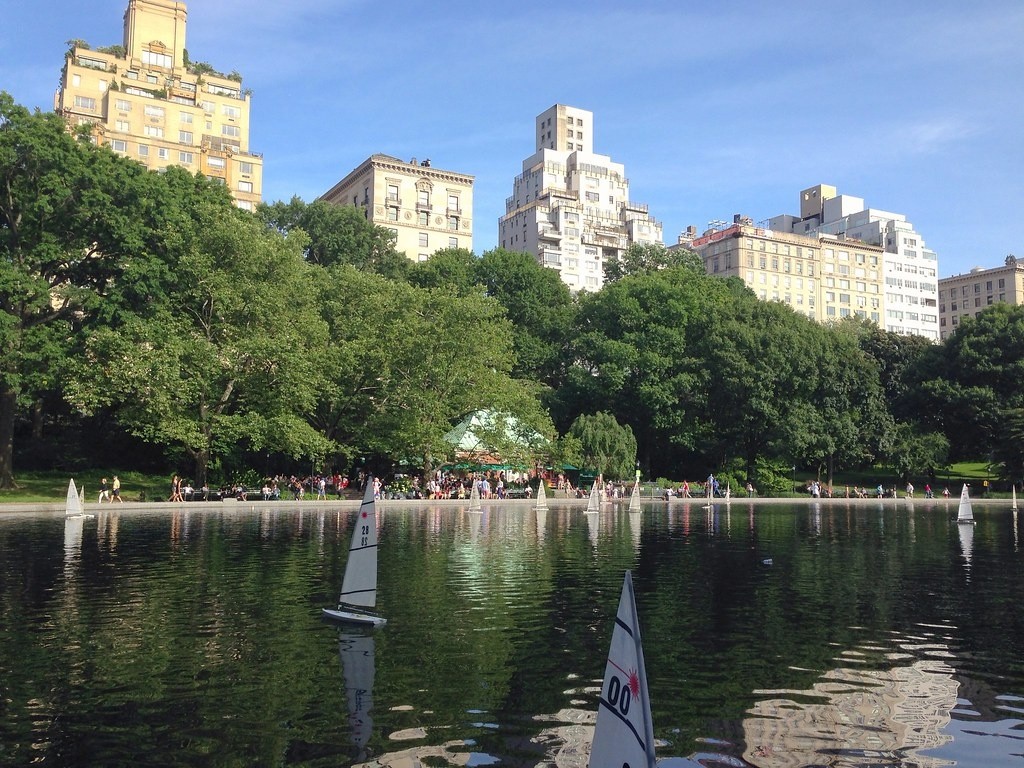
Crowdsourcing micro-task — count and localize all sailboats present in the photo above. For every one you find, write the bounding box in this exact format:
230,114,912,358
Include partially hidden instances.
535,511,549,552
321,470,389,625
531,478,549,511
65,477,95,519
628,512,644,559
467,514,482,547
958,525,976,584
1012,511,1020,552
462,478,483,514
585,515,601,565
336,627,378,764
955,481,977,525
64,520,84,583
701,480,730,509
1010,485,1020,511
588,567,660,767
622,477,644,513
582,478,600,514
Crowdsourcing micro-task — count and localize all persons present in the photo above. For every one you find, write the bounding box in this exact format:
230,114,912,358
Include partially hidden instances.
93,465,994,505
